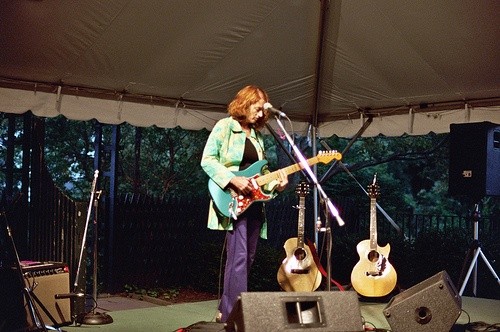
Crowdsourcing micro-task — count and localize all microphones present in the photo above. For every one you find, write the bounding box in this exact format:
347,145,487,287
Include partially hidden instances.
55,293,89,299
264,103,288,119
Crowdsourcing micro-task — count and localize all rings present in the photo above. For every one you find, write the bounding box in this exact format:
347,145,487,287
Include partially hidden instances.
280,181,283,182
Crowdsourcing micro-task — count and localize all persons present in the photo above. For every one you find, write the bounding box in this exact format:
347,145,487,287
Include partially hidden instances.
201,85,289,324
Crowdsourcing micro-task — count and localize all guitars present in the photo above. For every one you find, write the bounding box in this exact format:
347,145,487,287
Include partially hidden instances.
276,180,323,292
208,149,342,219
350,170,398,298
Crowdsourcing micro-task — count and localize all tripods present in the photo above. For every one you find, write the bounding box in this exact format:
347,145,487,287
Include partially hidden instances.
458,198,500,298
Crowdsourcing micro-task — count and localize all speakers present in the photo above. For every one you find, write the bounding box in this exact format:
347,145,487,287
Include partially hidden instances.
21,261,73,332
448,121,500,199
224,291,364,332
383,270,462,332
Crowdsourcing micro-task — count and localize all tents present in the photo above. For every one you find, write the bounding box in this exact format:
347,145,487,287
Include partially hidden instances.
0,0,500,253
